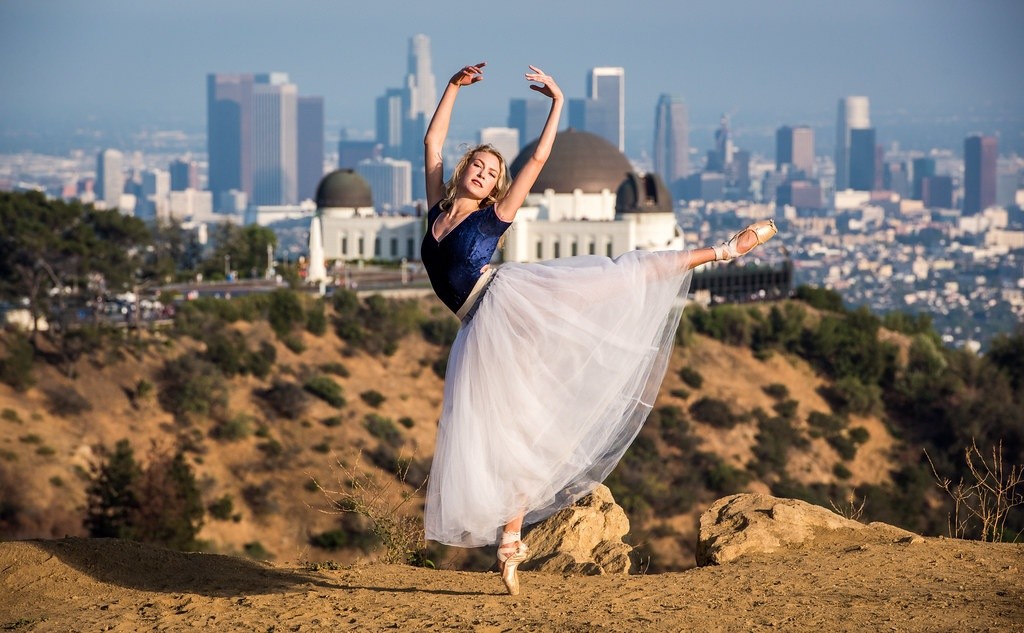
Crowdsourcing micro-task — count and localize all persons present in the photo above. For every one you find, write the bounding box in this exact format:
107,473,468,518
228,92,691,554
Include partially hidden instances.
267,254,352,295
421,63,779,597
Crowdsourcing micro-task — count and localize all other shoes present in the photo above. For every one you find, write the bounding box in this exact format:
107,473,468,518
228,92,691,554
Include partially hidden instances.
711,219,778,264
496,530,534,596
